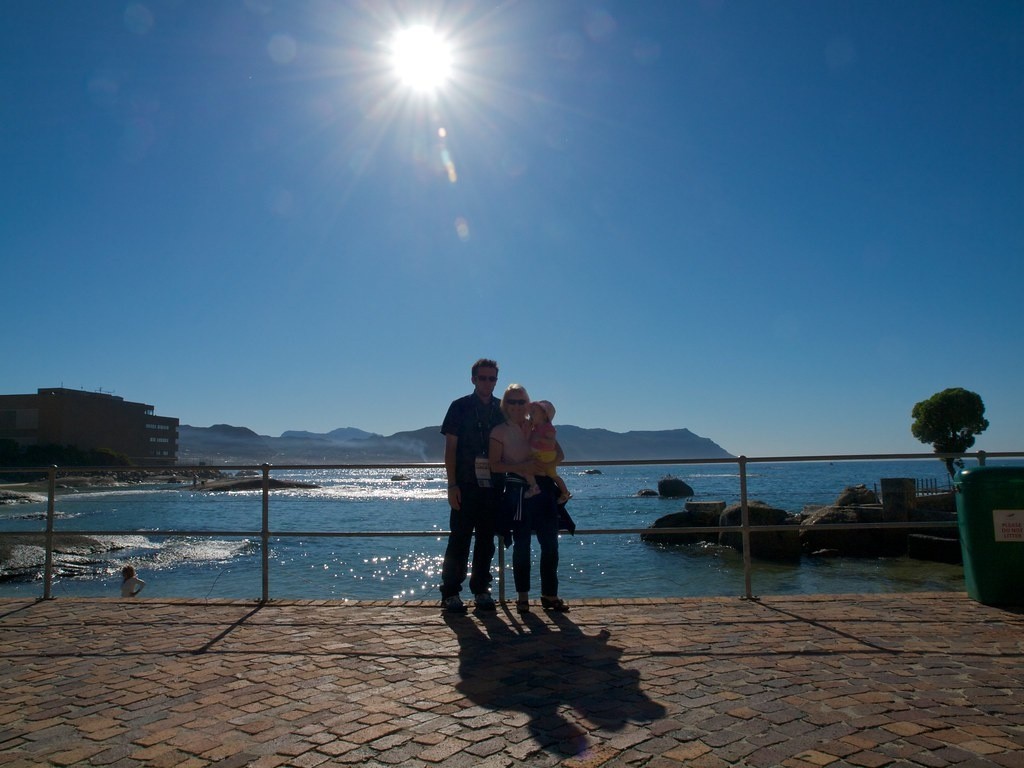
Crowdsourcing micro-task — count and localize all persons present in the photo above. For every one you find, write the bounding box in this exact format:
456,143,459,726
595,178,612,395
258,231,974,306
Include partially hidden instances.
192,470,199,488
489,384,576,614
440,359,508,617
119,566,145,597
522,400,572,505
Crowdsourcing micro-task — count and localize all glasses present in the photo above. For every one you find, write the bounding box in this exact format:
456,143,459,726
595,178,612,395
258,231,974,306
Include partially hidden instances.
505,399,526,405
475,375,498,381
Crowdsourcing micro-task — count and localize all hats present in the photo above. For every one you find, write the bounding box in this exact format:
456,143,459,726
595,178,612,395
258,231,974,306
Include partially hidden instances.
528,400,556,422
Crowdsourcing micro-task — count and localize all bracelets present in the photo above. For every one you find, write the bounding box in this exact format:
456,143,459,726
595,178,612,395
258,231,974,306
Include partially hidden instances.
448,484,457,489
522,465,527,473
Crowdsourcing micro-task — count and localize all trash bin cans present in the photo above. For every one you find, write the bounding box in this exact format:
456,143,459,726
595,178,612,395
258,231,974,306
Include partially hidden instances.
950,465,1024,614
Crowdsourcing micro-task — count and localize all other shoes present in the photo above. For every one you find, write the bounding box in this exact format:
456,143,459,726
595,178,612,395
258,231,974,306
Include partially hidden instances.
441,594,468,612
515,599,529,614
540,596,569,612
474,593,496,612
